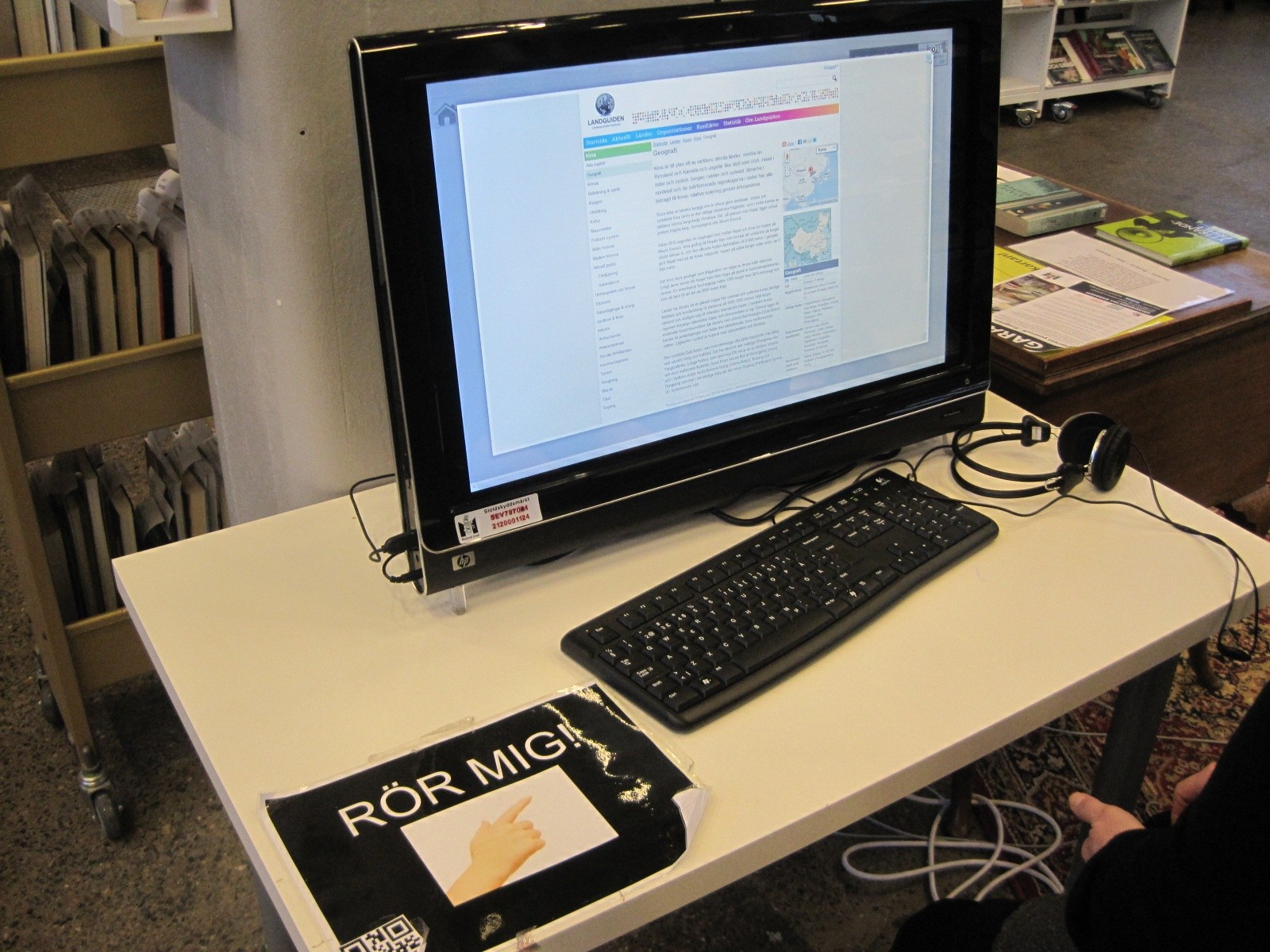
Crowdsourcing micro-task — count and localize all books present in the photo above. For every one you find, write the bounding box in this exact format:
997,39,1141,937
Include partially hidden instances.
1044,20,1176,87
0,0,223,627
995,174,1109,236
992,229,1253,376
1003,0,1056,10
1092,210,1250,266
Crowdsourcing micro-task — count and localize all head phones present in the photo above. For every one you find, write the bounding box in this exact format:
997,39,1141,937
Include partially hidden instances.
950,409,1137,499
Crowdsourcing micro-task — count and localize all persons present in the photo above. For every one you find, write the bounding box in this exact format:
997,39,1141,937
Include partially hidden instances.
447,794,545,906
893,678,1268,951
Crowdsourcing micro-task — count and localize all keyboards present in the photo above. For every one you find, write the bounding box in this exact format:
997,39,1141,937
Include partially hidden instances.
561,466,1000,734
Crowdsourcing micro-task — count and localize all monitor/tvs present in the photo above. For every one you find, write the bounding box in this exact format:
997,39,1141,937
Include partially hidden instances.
347,0,1004,596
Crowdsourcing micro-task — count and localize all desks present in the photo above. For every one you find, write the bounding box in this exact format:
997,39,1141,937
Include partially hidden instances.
112,393,1269,952
1000,2,1190,124
992,161,1270,502
